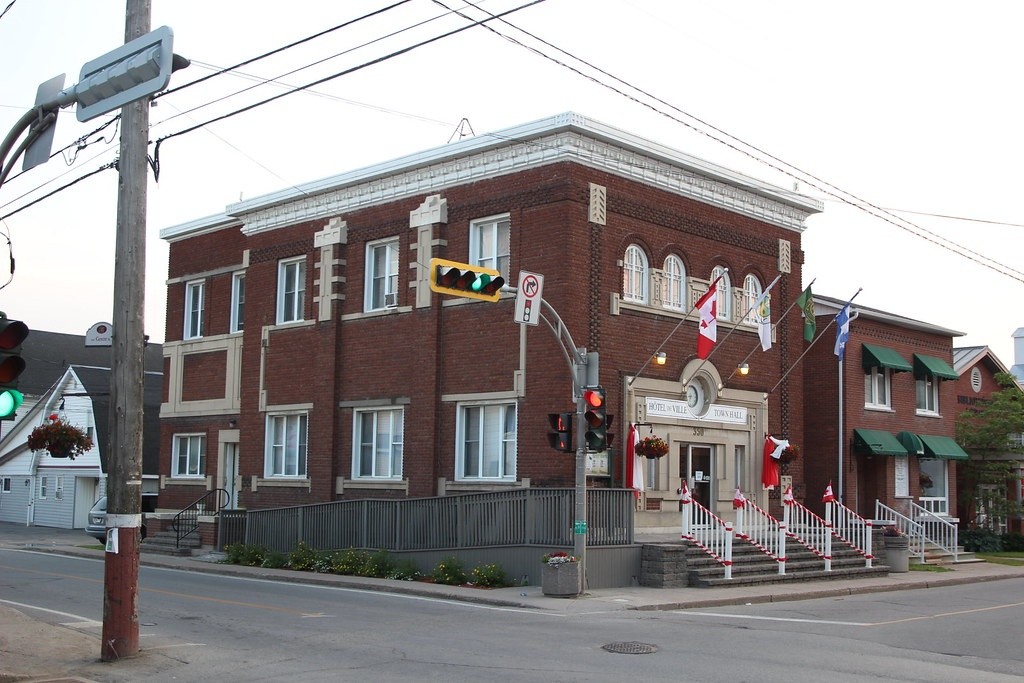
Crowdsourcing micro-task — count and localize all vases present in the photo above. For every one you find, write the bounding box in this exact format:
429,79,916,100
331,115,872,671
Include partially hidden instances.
646,455,655,460
49,442,71,458
780,455,792,463
542,562,581,597
884,536,909,573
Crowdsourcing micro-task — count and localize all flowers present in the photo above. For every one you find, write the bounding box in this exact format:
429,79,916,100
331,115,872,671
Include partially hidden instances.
771,444,800,463
635,435,669,460
881,525,906,537
543,552,581,569
27,414,93,461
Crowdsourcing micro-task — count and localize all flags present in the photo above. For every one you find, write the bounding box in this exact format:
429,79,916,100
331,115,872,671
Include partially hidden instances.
795,285,816,344
695,281,716,360
751,289,771,352
834,302,851,360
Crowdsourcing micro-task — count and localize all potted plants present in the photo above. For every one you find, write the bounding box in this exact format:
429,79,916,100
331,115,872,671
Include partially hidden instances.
196,498,206,509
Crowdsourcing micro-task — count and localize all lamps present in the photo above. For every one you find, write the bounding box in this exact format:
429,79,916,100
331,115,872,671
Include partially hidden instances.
737,363,749,374
654,351,666,364
228,419,236,428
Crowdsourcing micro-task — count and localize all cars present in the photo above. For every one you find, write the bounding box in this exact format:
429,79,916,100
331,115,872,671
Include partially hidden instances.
84,492,159,546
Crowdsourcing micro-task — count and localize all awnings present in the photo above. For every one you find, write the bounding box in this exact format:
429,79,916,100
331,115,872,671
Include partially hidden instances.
854,428,909,458
896,431,925,456
916,434,968,461
862,342,912,373
913,352,960,382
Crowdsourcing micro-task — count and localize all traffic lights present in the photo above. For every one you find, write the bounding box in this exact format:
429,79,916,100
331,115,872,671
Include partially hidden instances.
429,258,507,303
546,413,573,452
0,310,30,421
605,413,615,449
582,386,607,453
